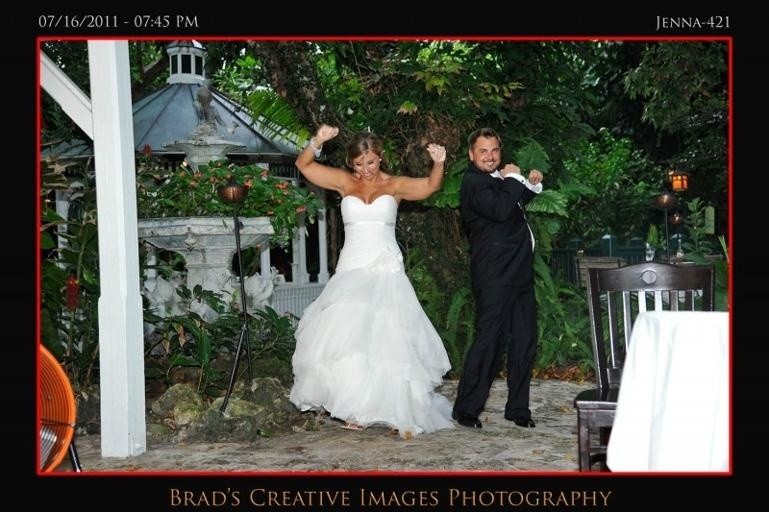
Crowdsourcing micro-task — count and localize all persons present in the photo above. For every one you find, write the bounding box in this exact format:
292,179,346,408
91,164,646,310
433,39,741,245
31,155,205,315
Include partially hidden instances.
289,123,454,439
451,128,545,429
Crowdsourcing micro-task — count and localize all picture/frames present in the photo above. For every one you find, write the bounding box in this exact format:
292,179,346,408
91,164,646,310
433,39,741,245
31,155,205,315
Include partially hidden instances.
0,0,769,512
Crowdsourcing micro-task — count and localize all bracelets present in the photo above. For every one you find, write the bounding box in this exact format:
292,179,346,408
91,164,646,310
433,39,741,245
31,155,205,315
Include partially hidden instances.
309,137,323,158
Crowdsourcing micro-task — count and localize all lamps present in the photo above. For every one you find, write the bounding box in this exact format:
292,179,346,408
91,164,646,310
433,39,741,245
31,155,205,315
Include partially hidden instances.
217,174,254,417
653,184,679,266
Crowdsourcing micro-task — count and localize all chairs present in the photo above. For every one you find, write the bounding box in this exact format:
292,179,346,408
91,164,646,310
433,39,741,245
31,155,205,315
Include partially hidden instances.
571,261,717,472
37,340,84,474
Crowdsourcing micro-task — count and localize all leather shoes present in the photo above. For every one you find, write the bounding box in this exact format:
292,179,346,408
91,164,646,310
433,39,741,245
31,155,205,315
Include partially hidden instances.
452,410,482,428
504,415,536,428
